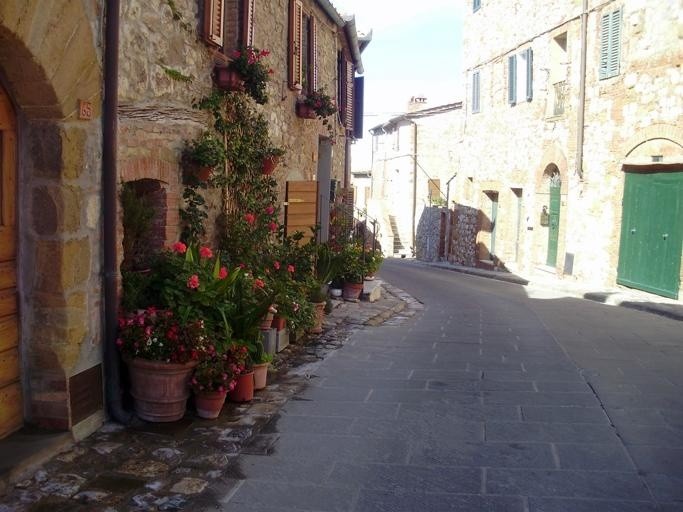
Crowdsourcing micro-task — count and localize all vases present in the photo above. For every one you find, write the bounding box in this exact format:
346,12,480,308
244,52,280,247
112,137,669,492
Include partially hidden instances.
213,66,246,90
123,351,271,420
297,101,318,118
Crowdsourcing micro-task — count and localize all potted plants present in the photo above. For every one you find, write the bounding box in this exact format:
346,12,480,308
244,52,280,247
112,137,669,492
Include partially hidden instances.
178,134,284,186
307,235,384,333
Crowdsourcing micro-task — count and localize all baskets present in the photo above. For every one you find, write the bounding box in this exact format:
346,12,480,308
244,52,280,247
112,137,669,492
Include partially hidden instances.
305,301,326,333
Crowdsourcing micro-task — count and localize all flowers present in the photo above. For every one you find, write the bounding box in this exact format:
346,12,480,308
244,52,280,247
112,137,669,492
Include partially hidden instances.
113,206,295,396
306,91,336,145
231,46,274,103
